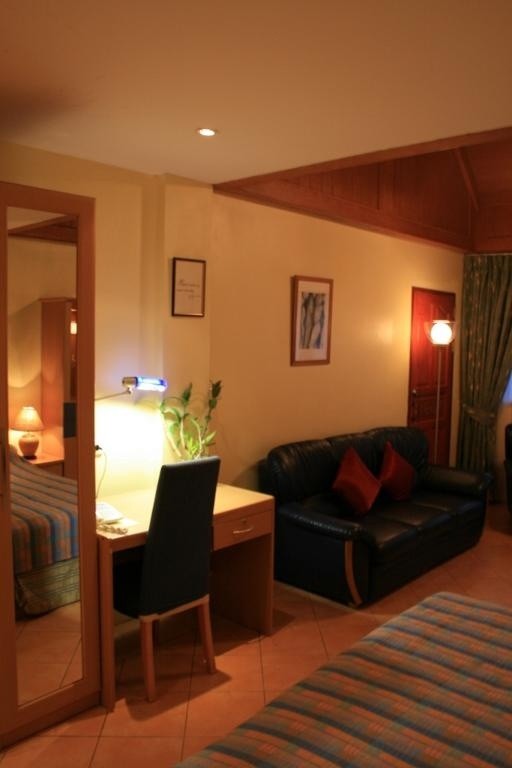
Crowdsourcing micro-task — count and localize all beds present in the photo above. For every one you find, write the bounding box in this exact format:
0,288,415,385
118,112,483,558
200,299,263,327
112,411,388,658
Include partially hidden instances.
12,446,77,619
174,592,512,768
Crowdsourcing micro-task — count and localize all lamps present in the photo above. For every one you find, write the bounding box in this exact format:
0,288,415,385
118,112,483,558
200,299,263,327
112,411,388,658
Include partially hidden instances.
95,375,168,402
432,320,453,465
11,404,44,459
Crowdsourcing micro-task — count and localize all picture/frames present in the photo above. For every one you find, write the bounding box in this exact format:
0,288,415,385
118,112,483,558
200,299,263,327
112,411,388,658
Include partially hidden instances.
172,257,206,317
290,275,334,366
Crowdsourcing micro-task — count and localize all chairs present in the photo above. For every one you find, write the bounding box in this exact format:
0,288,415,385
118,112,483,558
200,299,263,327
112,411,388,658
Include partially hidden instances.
113,456,221,702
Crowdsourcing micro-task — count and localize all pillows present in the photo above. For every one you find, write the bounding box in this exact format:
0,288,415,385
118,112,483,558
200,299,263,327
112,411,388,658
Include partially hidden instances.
330,442,418,518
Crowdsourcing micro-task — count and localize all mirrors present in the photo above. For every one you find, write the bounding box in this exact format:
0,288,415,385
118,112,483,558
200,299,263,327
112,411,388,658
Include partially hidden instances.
2,181,96,748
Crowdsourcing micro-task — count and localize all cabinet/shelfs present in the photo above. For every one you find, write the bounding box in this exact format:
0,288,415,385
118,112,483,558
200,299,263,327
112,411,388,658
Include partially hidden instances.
39,296,81,477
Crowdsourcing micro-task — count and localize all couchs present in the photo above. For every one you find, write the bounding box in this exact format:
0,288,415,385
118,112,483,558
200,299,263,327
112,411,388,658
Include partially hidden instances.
258,426,488,609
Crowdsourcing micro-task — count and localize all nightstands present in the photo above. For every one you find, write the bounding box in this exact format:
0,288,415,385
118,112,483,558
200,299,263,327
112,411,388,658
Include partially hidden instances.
25,455,64,477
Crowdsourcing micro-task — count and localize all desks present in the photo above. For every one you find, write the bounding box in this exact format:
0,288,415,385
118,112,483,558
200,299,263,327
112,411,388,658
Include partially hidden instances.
96,482,275,713
